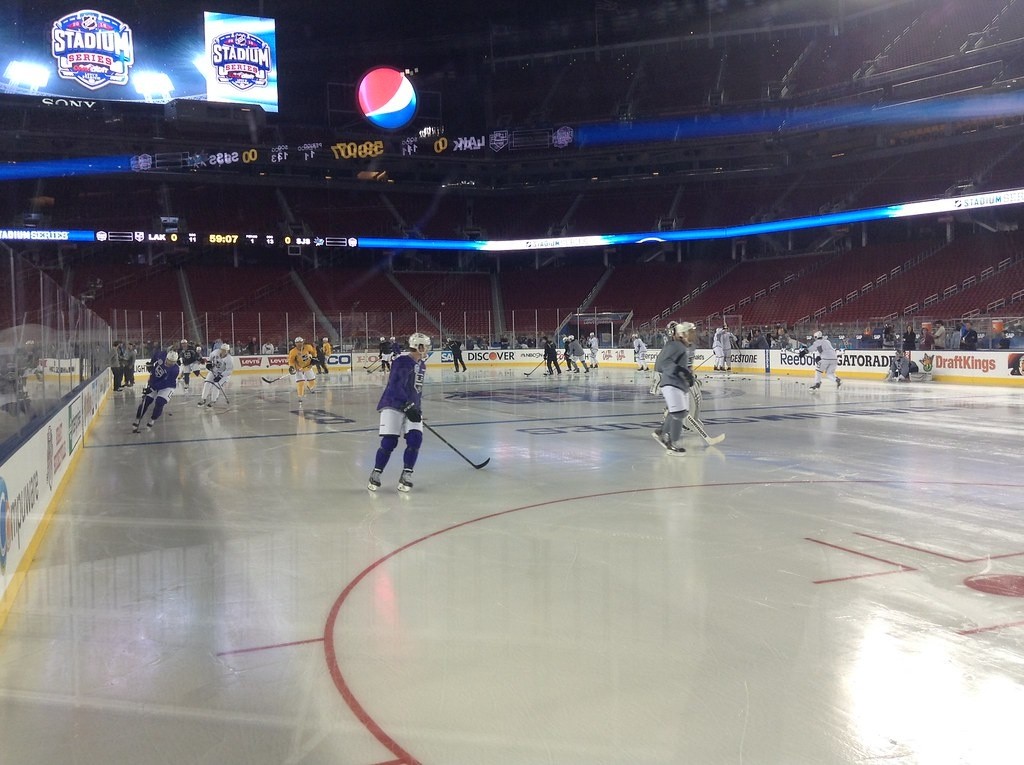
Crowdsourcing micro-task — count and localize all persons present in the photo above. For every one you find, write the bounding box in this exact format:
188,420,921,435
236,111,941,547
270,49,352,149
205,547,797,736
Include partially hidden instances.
112,320,1024,407
632,333,649,371
21,333,90,372
799,331,841,388
132,349,180,430
367,332,431,488
651,322,696,453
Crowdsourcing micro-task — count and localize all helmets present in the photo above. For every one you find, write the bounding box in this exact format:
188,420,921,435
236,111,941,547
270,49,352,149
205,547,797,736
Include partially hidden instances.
167,338,230,362
814,331,822,338
542,320,729,341
295,336,431,354
447,336,451,341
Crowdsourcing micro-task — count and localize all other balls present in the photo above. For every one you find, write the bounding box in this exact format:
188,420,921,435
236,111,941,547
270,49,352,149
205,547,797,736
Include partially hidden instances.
777,372,805,385
607,377,608,378
527,375,528,377
705,371,751,383
480,364,509,374
169,414,172,416
630,380,633,382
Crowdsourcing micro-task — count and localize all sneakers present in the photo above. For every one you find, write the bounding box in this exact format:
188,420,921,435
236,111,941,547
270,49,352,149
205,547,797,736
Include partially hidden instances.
132,418,155,433
836,377,842,386
809,383,820,390
397,468,413,492
367,468,384,491
651,429,686,457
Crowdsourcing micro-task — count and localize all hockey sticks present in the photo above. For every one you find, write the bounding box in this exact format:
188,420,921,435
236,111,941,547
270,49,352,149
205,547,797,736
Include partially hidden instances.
136,370,151,433
523,359,546,375
543,355,565,376
686,414,725,446
786,347,816,360
363,359,378,369
210,368,229,404
367,364,382,373
423,422,490,469
734,342,742,355
262,364,311,383
694,354,714,370
199,373,206,379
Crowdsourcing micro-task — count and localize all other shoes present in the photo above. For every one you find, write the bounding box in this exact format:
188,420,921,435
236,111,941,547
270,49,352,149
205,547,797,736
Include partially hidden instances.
113,382,216,409
566,363,598,375
713,365,719,372
307,385,315,393
638,366,650,370
727,366,732,372
720,367,726,372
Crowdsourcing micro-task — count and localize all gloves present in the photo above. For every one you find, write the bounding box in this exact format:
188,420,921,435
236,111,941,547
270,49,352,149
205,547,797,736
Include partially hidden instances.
289,357,320,374
145,361,154,372
800,351,805,357
214,374,223,381
402,402,422,423
816,356,821,363
206,363,212,370
673,364,693,386
143,386,156,394
543,352,570,359
